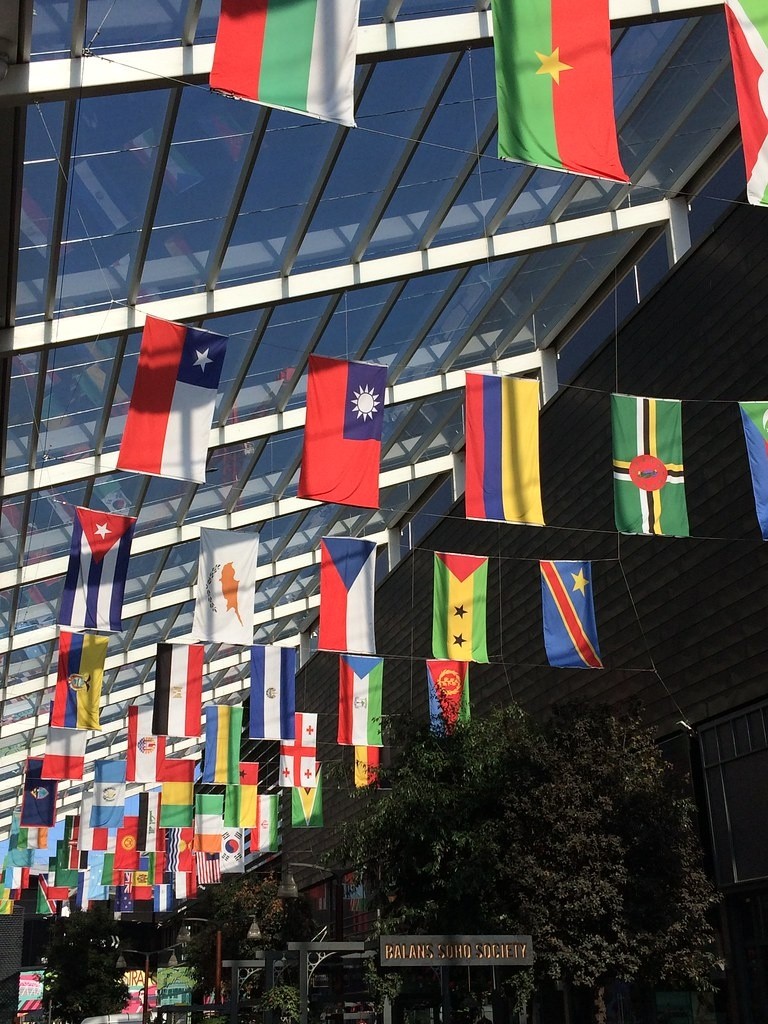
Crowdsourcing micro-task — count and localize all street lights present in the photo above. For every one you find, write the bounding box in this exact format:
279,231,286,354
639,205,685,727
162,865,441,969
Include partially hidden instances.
115,946,179,1024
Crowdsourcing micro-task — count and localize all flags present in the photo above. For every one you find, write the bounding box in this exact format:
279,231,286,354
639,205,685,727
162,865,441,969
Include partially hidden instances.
250,645,296,739
209,0,358,132
319,538,383,746
725,0,768,207
465,370,546,528
49,506,135,731
1,701,394,930
738,401,768,541
117,315,229,484
6,334,133,547
1,581,46,664
298,350,387,511
427,550,490,733
491,0,633,187
147,524,261,737
608,393,692,538
19,105,245,263
538,560,604,668
